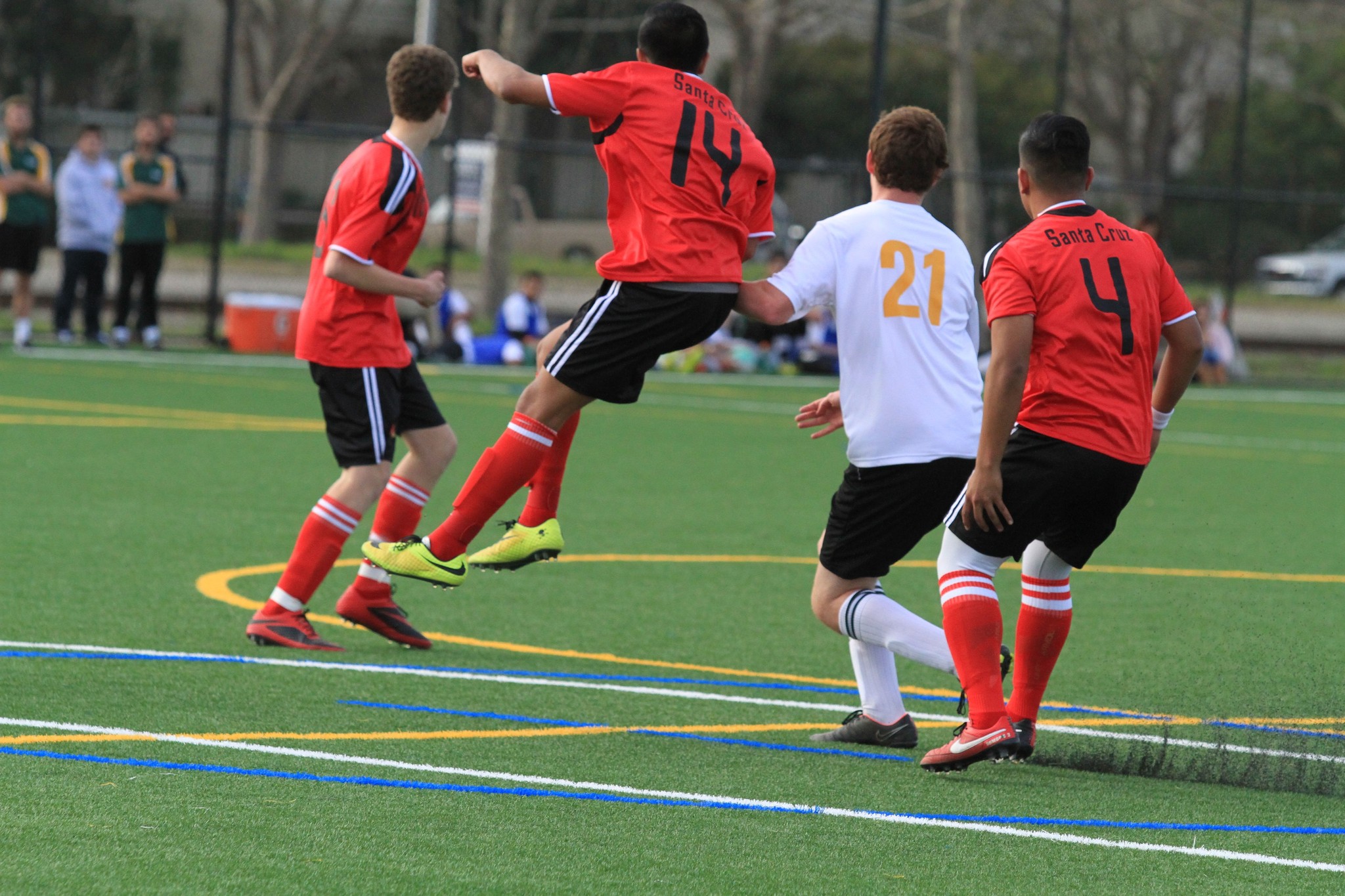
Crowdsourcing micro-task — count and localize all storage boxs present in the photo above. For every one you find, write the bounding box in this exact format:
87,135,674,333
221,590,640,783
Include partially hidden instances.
223,292,304,355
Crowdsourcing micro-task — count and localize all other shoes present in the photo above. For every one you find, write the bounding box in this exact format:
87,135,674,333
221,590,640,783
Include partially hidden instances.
13,318,161,348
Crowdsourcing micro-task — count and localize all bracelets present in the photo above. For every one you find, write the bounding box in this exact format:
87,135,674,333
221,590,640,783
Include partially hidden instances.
1151,406,1176,431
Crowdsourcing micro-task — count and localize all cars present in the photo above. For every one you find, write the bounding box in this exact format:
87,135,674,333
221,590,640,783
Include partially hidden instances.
421,180,616,264
1254,222,1345,298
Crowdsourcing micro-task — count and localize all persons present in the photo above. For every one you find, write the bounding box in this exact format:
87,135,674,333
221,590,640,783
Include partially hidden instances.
1188,297,1238,388
52,121,126,349
720,246,808,365
359,0,777,589
127,104,190,343
0,94,55,350
916,110,1206,775
400,261,478,364
245,42,460,650
802,300,842,378
727,102,1014,751
474,267,553,369
1136,211,1175,264
113,113,183,354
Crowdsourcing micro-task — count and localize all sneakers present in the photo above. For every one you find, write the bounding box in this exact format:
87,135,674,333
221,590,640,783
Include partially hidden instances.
987,718,1036,764
919,716,1018,773
810,713,918,749
361,539,469,588
466,518,565,571
336,585,432,649
246,610,346,652
